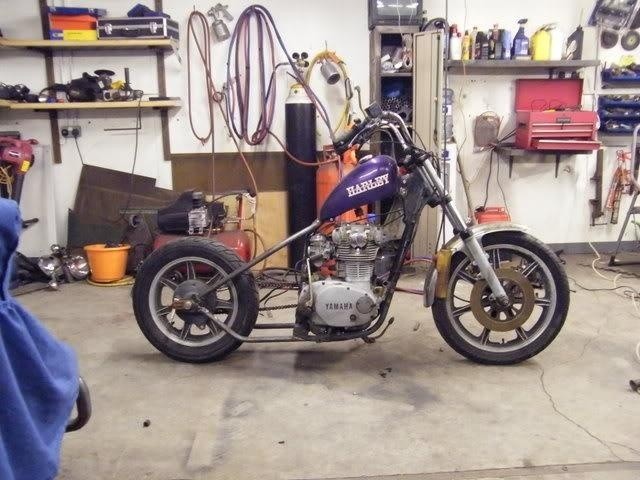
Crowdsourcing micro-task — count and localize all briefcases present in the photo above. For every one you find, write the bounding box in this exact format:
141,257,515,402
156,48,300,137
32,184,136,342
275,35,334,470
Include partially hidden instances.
97,17,179,40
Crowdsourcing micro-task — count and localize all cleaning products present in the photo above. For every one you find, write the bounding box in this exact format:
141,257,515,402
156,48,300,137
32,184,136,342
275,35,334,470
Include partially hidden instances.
449,24,462,60
512,18,529,57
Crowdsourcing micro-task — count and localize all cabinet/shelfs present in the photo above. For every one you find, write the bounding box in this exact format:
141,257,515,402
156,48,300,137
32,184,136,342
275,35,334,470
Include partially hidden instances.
0,38,183,112
368,24,640,277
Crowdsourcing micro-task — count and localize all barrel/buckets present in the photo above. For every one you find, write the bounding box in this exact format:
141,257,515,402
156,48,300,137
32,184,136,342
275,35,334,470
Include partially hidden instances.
83,243,133,283
549,26,564,62
474,206,510,224
530,29,550,60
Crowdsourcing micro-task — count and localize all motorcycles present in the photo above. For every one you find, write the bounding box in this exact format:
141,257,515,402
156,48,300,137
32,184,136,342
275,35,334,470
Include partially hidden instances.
133,103,569,365
